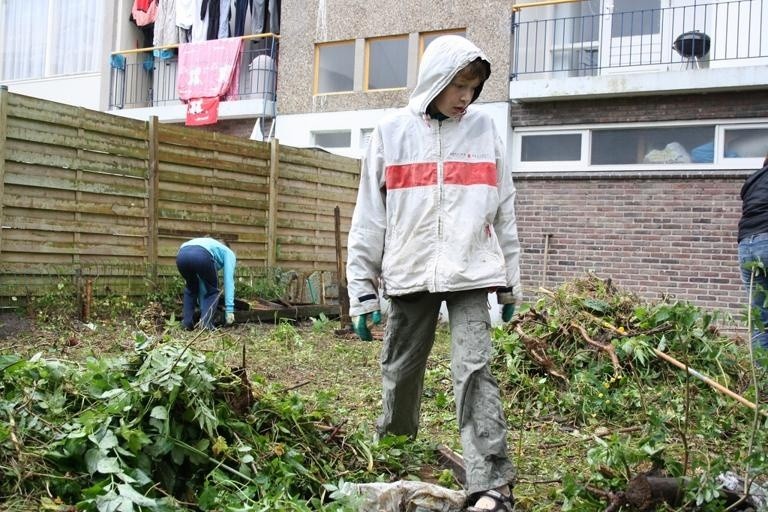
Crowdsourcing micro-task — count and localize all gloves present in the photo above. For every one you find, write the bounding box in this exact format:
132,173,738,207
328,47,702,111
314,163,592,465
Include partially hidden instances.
225,312,234,324
352,311,381,341
502,304,514,322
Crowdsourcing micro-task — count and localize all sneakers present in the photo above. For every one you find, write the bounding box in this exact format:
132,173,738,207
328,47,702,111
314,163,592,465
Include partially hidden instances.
459,484,515,512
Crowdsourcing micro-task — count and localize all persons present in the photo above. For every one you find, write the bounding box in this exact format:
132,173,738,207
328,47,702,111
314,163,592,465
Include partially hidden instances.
736,156,768,406
175,236,237,331
344,31,525,512
